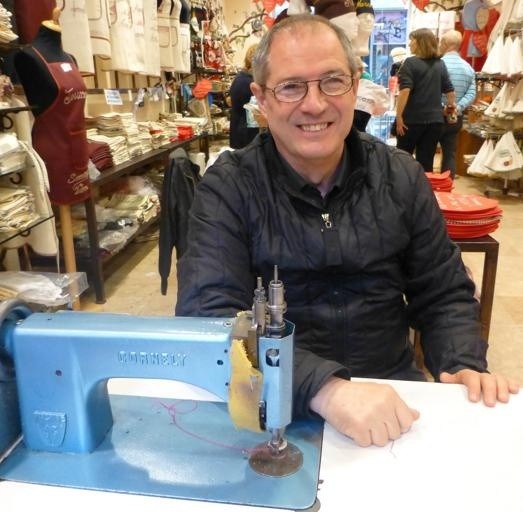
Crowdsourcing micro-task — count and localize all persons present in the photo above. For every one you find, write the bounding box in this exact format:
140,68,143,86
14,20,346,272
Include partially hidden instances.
392,28,456,172
352,0,374,56
389,61,401,111
12,22,93,201
314,0,360,41
358,60,372,133
173,11,517,445
429,28,476,182
229,44,259,150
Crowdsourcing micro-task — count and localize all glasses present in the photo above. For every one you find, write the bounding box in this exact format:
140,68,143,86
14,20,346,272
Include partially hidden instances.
266,72,356,103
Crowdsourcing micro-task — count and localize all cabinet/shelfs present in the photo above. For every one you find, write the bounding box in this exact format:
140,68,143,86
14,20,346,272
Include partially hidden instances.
0,102,211,311
165,67,237,118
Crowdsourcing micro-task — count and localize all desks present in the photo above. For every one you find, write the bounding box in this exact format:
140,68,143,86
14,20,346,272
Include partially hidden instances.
412,234,499,370
1,375,523,512
